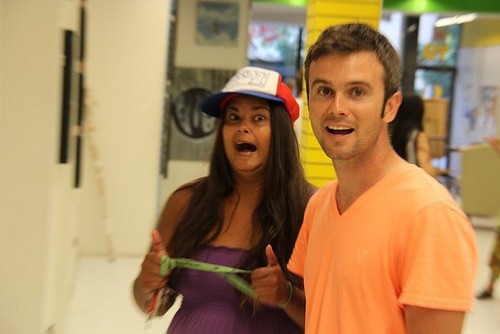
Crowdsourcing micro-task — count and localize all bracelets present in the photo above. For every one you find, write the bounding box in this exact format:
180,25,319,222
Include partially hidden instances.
278,280,293,307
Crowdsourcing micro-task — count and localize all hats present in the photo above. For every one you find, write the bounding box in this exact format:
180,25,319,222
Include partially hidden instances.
201,66,299,124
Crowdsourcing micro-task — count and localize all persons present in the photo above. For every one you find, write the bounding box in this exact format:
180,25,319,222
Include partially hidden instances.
133,66,320,334
286,24,478,334
391,93,437,178
476,229,500,299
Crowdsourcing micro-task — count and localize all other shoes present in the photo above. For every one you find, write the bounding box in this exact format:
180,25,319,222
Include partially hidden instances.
477,290,493,299
